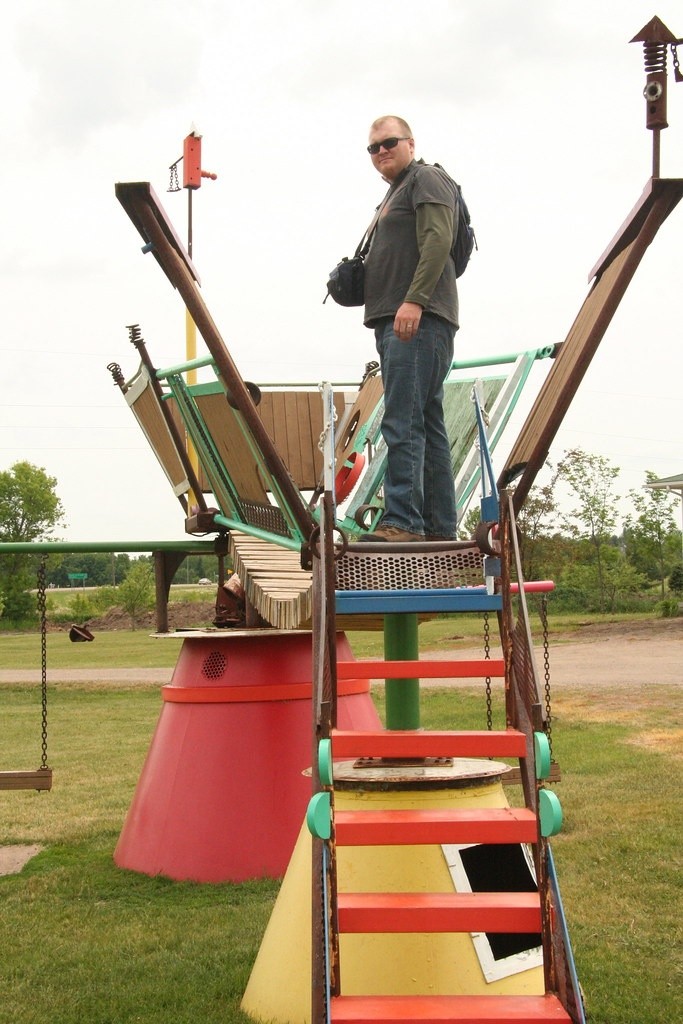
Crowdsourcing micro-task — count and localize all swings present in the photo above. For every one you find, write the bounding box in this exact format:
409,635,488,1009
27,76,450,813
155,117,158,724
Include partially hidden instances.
0,552,54,791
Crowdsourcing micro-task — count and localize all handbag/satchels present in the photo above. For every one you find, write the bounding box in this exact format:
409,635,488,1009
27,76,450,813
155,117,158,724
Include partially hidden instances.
322,256,364,307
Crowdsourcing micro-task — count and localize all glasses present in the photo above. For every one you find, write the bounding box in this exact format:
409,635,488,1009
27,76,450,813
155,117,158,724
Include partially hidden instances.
367,137,410,154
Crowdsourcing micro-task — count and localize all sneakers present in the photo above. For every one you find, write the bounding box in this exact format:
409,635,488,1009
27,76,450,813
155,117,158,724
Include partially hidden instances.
425,535,457,541
359,525,425,542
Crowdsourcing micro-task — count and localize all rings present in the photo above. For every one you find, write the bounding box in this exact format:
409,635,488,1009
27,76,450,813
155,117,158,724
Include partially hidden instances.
407,324,412,328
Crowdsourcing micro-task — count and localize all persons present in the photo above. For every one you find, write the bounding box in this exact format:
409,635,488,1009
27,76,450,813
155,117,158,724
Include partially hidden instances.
357,115,458,542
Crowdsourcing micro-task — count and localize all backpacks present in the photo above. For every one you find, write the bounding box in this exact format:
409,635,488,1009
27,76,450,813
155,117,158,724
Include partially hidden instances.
407,163,478,278
